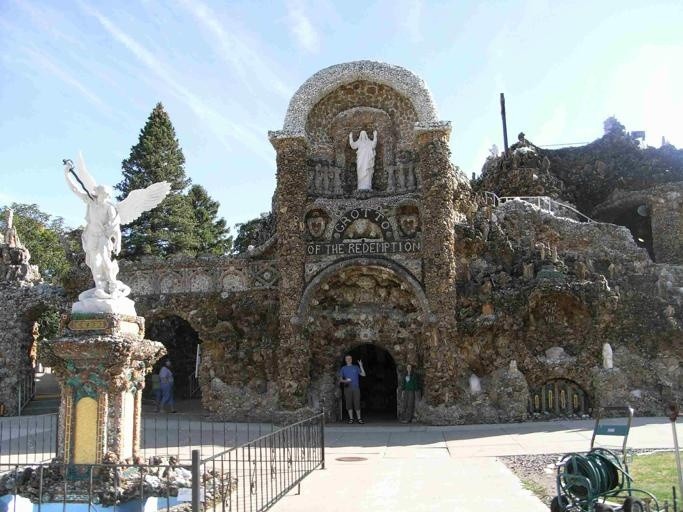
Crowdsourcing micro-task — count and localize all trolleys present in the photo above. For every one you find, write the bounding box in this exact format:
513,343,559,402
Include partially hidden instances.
550,405,646,512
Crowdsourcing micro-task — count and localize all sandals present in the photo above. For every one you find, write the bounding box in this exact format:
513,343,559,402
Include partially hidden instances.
347,418,355,425
357,418,365,424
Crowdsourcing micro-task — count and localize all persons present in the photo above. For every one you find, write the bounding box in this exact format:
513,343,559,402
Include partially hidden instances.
64,166,122,298
399,363,418,424
152,359,179,413
340,355,366,424
349,130,377,192
